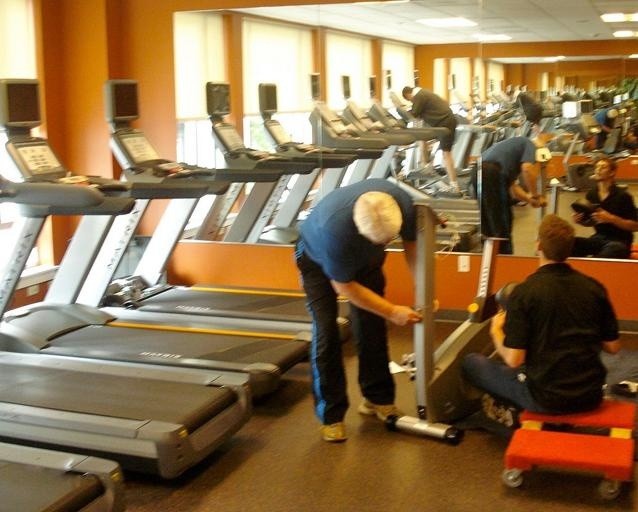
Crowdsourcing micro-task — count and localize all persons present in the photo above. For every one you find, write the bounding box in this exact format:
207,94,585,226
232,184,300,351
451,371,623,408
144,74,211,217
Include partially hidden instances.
462,213,622,428
567,155,637,259
293,177,441,444
402,86,462,197
503,82,637,158
469,136,549,257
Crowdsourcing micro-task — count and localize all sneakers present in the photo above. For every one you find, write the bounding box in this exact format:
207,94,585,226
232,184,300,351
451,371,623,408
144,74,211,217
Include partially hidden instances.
356,397,405,424
437,182,462,193
420,161,434,177
481,393,519,430
318,422,349,443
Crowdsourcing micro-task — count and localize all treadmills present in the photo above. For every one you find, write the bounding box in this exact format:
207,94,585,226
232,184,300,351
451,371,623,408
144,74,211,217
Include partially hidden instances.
0,443,125,512
309,73,485,231
252,81,482,252
2,78,319,405
194,80,458,253
337,74,534,237
39,78,354,343
0,179,257,490
